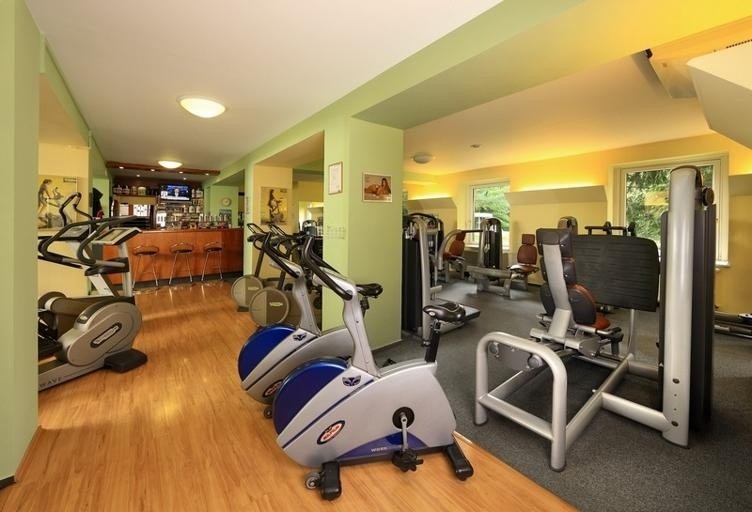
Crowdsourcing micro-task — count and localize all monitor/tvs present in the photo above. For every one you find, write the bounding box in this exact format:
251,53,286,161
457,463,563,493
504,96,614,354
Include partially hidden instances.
159,184,191,202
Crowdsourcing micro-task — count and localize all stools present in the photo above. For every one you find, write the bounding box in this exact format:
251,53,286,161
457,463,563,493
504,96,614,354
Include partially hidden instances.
131,242,224,292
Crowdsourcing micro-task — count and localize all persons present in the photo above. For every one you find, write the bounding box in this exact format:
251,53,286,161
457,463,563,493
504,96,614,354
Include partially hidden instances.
268,189,278,216
376,178,390,195
170,188,184,196
37,179,52,215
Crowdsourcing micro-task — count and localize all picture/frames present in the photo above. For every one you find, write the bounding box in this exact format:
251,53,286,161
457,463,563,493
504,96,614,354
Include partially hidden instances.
328,162,343,195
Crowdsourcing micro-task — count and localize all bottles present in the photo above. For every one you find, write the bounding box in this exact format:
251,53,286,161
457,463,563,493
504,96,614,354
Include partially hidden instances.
184,204,201,214
199,212,229,222
131,186,146,196
192,188,204,199
